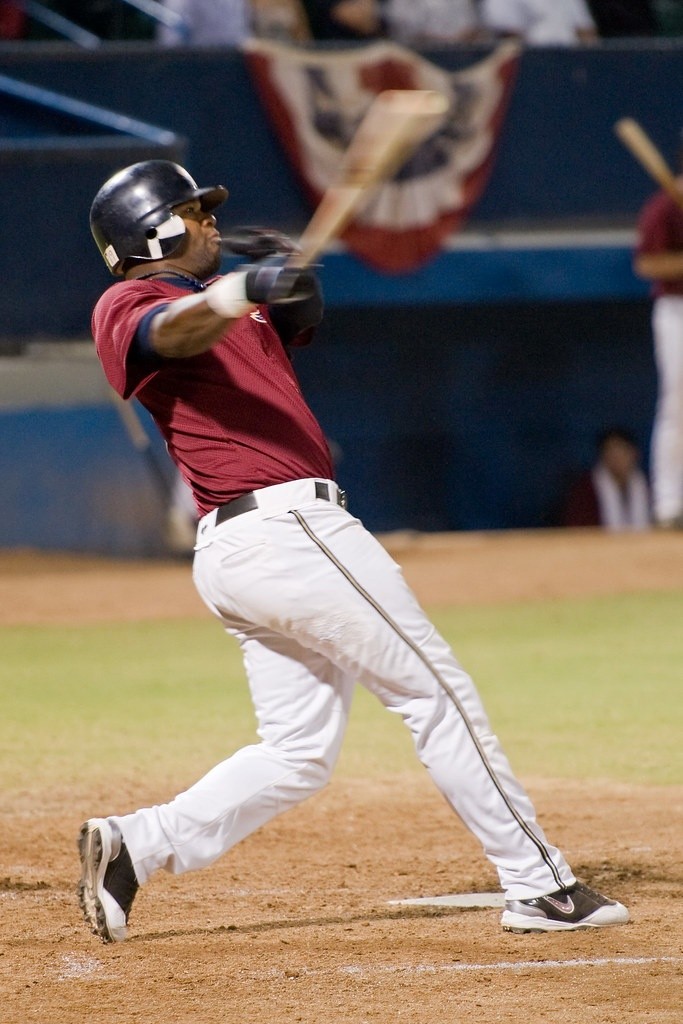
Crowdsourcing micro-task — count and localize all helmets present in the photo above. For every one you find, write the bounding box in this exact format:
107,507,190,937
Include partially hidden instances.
90,160,228,275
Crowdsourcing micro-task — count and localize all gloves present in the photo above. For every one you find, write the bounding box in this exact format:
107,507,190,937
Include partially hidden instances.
245,262,323,343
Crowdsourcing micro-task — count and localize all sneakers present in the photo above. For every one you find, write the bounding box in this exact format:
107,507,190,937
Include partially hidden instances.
76,817,139,942
501,881,630,935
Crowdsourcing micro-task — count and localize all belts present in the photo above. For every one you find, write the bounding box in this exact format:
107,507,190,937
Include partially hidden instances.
216,482,347,526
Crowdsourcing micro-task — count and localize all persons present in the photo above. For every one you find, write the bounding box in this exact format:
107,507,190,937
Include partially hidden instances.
155,0,598,48
76,159,631,945
634,168,683,532
581,429,654,533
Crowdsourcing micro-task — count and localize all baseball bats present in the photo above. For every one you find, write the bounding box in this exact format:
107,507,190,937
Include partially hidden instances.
295,88,450,268
613,116,683,208
